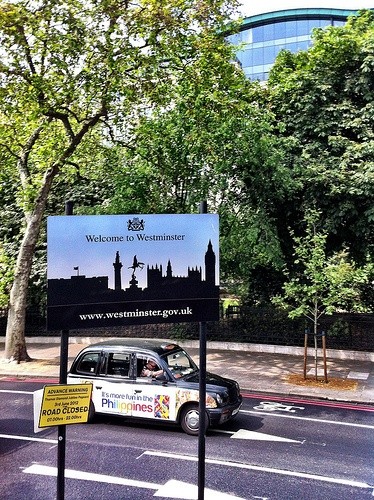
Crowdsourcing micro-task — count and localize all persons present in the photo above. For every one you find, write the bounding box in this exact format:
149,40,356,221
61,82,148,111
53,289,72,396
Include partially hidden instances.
141,358,163,378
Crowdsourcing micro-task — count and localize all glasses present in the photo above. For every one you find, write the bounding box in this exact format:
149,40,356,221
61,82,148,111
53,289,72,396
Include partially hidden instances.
149,363,156,368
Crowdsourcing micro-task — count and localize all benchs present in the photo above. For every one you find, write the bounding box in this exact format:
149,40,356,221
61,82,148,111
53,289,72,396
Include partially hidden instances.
81,358,98,372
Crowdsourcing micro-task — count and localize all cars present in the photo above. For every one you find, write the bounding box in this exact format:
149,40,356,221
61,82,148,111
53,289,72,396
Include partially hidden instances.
62,337,242,435
